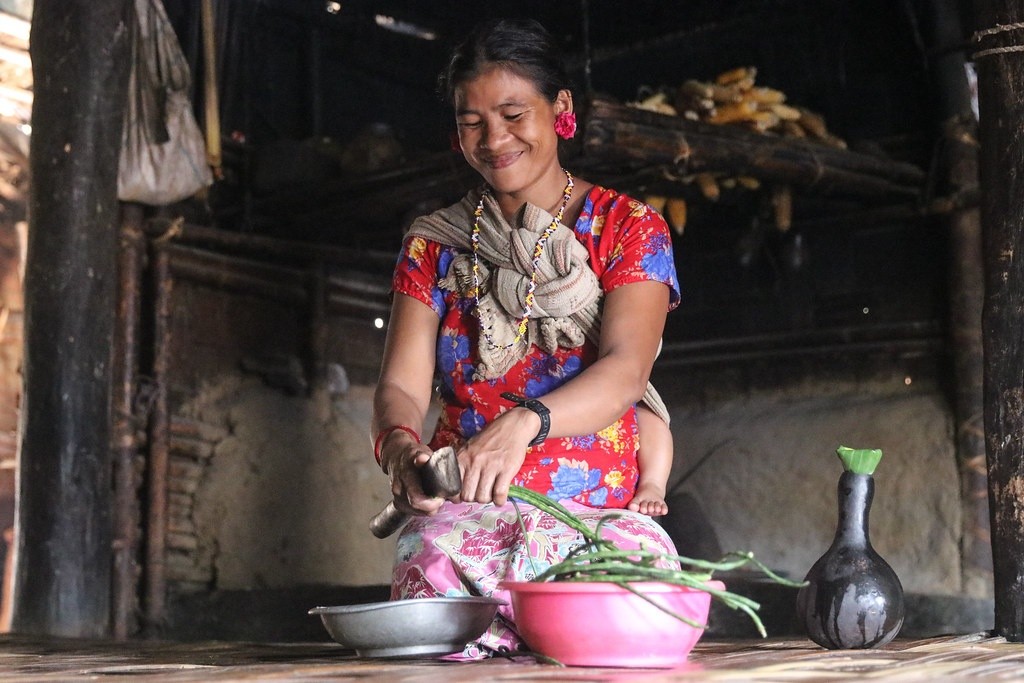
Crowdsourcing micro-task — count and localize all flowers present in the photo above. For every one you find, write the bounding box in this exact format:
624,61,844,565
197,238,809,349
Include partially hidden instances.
555,111,577,141
448,130,462,154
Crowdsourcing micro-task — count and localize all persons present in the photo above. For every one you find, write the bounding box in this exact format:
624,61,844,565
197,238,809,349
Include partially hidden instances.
370,15,681,662
628,403,673,516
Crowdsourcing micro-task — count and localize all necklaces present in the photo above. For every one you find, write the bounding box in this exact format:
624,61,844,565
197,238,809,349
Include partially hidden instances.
472,168,573,348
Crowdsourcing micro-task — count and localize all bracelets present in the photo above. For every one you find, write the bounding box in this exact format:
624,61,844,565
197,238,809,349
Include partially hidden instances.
374,425,421,467
501,392,551,447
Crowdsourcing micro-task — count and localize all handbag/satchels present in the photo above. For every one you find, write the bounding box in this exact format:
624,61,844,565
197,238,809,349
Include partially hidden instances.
117,0,214,204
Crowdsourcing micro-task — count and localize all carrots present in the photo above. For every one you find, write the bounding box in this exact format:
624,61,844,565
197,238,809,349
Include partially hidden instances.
643,66,826,236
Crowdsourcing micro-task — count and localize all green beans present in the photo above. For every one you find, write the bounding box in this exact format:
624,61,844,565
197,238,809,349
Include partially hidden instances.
508,484,810,638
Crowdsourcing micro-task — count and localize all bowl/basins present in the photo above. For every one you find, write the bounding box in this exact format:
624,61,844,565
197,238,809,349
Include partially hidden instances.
306,597,510,658
495,581,727,669
